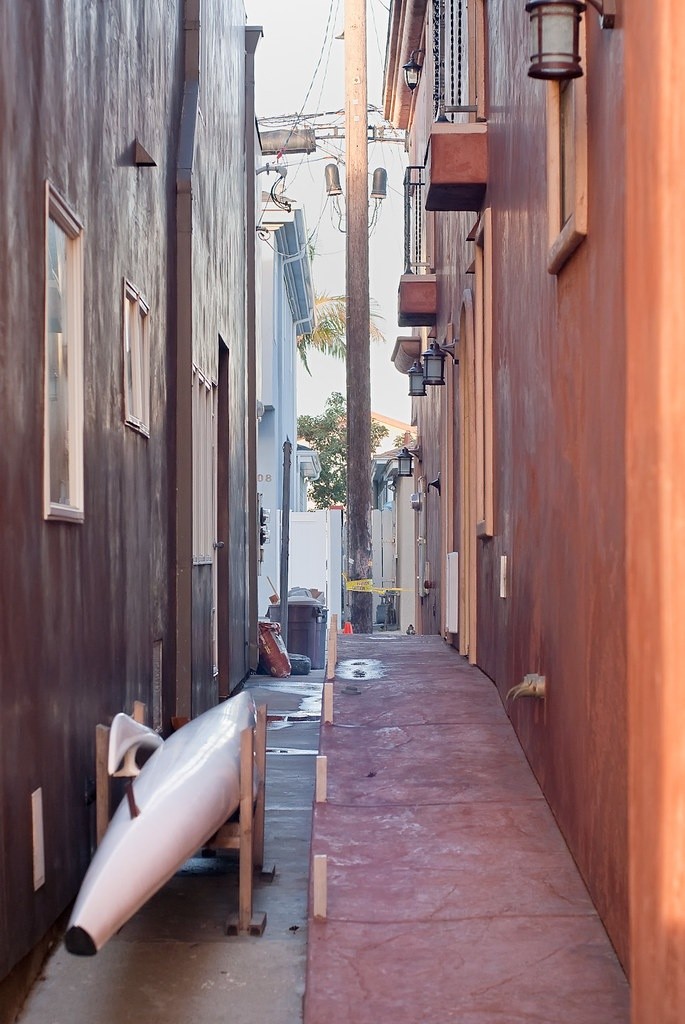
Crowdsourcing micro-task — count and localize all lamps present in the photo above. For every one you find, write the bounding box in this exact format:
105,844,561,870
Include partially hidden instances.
407,362,428,396
397,447,423,477
326,164,343,195
411,493,419,510
260,528,270,545
261,507,271,526
524,0,616,82
370,167,386,199
420,340,460,385
402,48,423,96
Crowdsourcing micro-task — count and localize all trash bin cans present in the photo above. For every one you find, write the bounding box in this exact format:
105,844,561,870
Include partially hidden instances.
376,594,386,624
266,596,330,669
